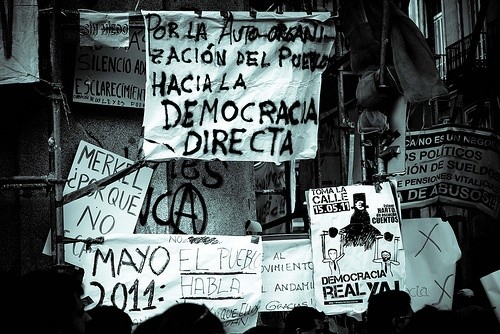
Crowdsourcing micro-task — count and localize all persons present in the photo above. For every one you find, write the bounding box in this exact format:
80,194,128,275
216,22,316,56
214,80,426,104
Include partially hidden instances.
12,270,89,334
85,290,500,334
